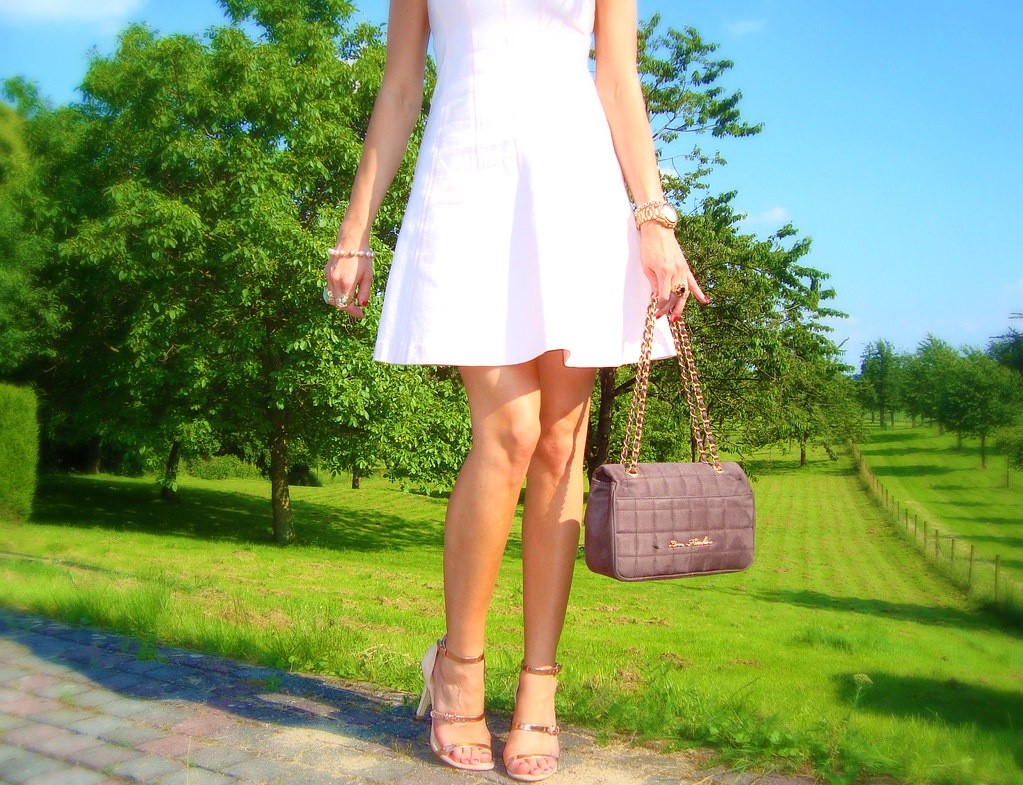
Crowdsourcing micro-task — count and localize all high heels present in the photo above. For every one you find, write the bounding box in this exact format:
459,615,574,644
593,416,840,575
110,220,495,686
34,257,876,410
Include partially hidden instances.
503,659,562,782
415,635,495,771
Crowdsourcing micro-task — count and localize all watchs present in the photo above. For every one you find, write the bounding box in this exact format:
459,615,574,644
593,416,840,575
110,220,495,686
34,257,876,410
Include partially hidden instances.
635,202,678,230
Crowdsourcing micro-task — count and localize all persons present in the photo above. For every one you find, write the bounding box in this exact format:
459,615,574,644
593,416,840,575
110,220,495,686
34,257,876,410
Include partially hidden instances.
323,0,712,783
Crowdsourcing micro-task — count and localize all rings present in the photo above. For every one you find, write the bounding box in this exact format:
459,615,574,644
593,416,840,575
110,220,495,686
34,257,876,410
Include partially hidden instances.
670,282,686,297
323,287,335,304
337,293,354,309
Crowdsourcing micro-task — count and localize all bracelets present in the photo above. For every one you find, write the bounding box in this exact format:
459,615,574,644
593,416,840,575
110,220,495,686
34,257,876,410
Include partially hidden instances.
327,248,374,258
633,200,666,214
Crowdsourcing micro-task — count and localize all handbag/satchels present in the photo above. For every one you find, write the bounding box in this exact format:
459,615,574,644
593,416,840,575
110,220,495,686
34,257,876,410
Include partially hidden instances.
582,293,755,583
